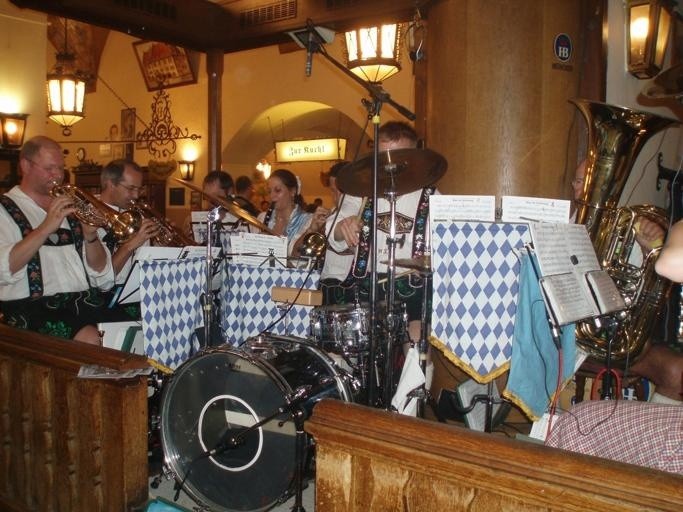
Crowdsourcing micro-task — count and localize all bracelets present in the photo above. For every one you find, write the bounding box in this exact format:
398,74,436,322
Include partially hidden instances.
82,236,99,243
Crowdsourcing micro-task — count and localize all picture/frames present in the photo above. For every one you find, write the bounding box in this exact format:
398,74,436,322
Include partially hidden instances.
130,38,197,93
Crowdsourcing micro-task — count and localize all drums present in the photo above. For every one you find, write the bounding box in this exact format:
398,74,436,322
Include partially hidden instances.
306,299,382,358
157,332,354,512
372,299,410,348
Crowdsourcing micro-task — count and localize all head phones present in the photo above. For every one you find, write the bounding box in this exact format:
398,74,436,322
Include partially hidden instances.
404,21,427,60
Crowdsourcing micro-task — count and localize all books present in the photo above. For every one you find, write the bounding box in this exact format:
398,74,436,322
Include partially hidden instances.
527,221,628,327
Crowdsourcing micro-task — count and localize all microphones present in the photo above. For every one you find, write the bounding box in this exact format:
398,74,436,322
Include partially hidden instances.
545,306,561,351
306,26,315,75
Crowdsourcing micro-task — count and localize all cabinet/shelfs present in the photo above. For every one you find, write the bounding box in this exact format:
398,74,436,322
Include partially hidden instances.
71,164,167,221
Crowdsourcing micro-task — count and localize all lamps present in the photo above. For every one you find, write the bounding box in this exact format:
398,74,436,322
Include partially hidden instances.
620,0,675,83
343,23,405,89
175,138,201,181
0,111,31,151
265,107,351,165
44,15,88,137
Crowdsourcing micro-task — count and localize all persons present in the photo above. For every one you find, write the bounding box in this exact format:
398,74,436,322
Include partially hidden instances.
543,214,683,474
569,152,664,267
90,158,162,322
0,133,116,348
324,119,451,286
180,160,358,279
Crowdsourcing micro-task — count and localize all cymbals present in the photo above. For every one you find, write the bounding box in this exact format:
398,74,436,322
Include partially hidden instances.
335,149,447,198
380,256,431,273
173,177,280,236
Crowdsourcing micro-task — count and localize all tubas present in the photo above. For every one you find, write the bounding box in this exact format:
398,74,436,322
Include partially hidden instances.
565,98,683,367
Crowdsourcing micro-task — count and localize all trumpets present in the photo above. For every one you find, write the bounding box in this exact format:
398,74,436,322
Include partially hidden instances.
129,199,199,247
48,179,142,240
304,206,336,251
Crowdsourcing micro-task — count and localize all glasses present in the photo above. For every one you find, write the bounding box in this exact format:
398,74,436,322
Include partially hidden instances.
118,183,146,195
25,157,54,174
